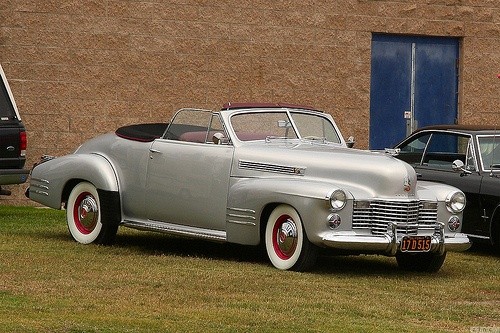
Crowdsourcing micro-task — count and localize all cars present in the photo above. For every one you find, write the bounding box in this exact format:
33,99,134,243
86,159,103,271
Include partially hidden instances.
0,64,30,196
392,124,500,256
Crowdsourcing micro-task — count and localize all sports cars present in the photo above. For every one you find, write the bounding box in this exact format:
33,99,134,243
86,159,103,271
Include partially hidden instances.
26,102,472,274
